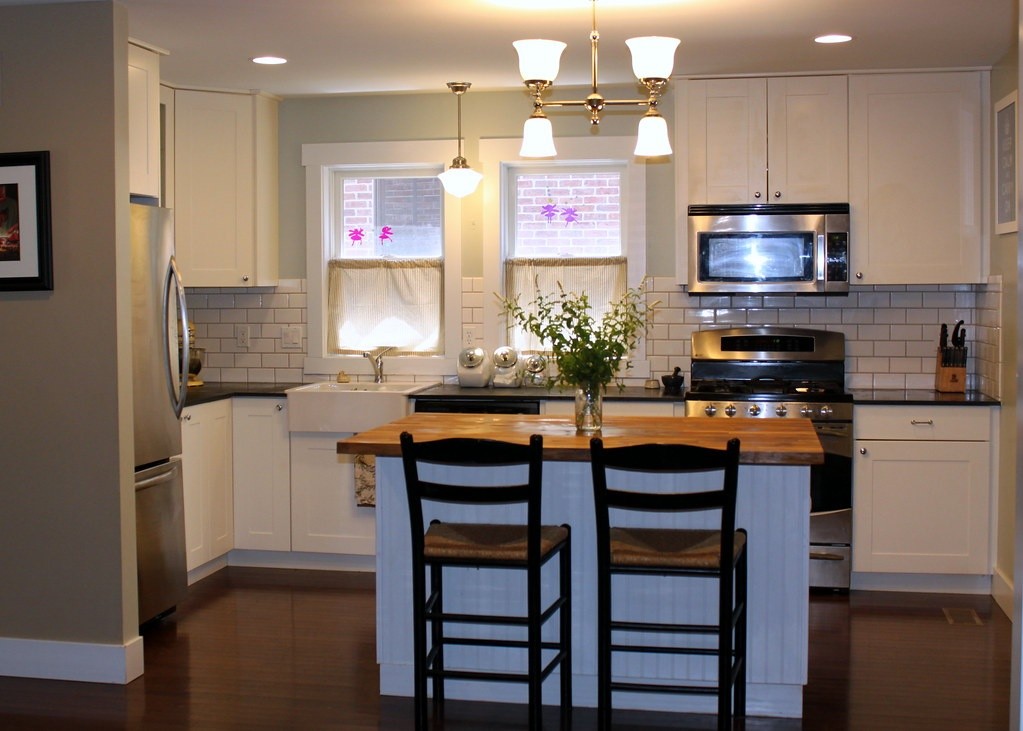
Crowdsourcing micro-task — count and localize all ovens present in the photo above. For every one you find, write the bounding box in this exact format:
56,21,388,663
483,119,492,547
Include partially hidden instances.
804,422,854,589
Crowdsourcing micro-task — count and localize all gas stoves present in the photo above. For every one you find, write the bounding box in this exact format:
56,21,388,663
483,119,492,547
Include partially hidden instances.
683,327,854,423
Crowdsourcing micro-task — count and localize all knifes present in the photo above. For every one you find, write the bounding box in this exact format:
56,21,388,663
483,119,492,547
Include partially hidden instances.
939,320,968,367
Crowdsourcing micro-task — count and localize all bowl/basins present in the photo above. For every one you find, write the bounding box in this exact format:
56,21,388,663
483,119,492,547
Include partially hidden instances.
179,348,205,376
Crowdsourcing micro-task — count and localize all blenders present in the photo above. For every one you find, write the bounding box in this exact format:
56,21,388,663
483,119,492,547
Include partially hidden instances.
177,318,204,386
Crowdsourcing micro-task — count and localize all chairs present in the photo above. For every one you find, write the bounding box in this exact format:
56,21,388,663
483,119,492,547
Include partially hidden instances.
588,435,748,731
400,431,572,731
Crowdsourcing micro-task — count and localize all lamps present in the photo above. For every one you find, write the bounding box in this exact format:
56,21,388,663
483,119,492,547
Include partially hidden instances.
511,0,682,158
437,80,485,200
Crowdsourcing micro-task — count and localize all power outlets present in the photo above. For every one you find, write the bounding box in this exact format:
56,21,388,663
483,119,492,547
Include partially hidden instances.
462,326,476,349
237,324,252,347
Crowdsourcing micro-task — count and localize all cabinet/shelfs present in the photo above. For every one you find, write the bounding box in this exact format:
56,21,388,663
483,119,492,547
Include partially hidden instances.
853,404,997,609
687,79,987,285
180,396,377,585
172,89,280,289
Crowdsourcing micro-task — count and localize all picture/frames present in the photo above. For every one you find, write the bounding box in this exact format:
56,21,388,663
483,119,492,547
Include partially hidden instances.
1,149,55,292
992,88,1019,235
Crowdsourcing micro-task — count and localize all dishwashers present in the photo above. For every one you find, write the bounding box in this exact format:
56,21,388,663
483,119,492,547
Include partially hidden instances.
415,401,539,416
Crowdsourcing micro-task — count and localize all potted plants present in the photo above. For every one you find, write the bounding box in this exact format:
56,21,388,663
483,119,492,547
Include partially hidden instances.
492,270,665,432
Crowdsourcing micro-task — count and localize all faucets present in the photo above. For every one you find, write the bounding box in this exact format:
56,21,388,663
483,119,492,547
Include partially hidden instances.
362,347,393,383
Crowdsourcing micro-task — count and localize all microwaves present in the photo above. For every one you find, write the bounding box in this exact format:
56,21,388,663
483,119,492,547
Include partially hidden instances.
687,204,851,296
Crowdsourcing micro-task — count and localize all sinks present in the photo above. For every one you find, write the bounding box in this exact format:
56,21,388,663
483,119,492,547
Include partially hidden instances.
284,380,441,432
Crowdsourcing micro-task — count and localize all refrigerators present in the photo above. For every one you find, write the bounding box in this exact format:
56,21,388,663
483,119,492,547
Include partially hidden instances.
126,201,191,629
